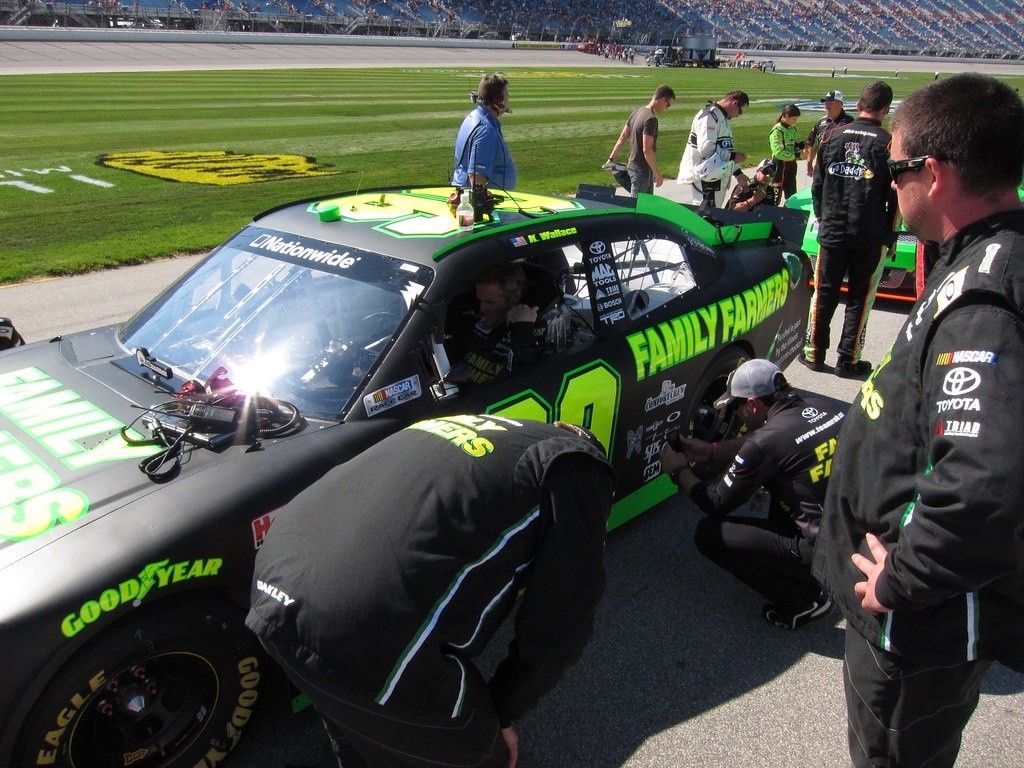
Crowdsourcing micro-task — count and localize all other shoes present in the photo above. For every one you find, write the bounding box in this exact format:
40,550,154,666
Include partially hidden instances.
834,354,871,377
796,355,824,372
602,163,627,174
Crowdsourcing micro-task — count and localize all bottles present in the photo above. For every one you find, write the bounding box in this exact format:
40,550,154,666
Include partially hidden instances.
456,194,474,231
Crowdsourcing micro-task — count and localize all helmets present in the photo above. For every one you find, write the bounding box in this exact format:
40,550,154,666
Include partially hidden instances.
694,144,734,183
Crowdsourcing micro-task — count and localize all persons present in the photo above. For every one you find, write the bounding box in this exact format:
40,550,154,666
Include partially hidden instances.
807,70,1024,768
796,78,905,380
600,83,675,202
0,1,1024,78
461,262,550,398
895,70,899,78
675,89,856,214
934,70,942,80
658,355,848,632
450,72,517,194
239,413,619,768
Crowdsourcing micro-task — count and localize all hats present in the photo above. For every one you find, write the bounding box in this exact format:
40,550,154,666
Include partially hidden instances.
713,359,786,410
568,424,606,458
820,90,844,104
757,159,776,171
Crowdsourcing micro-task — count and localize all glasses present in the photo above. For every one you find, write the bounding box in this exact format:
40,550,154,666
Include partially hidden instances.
887,155,946,184
733,96,743,115
661,95,671,109
760,167,774,177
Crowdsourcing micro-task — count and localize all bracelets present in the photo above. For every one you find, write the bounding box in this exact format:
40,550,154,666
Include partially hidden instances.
607,157,613,161
668,465,692,485
711,441,718,463
746,199,752,209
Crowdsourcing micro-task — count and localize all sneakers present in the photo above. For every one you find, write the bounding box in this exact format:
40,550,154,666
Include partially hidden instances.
762,585,837,629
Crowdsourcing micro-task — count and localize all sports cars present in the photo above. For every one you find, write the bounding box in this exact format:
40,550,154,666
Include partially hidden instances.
785,185,1024,305
0,184,818,768
